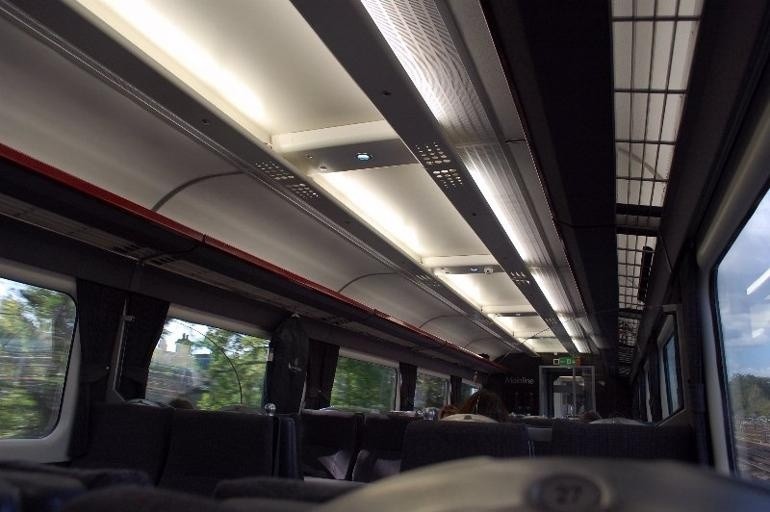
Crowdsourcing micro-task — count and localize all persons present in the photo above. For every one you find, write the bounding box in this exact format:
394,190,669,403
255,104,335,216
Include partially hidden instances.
456,386,513,423
